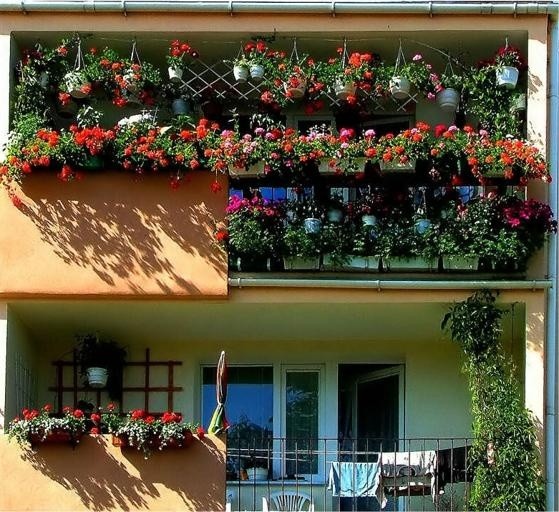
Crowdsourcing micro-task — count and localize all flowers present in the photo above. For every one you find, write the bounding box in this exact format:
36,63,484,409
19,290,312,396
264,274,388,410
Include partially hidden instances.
101,404,205,460
208,26,559,275
9,402,101,442
1,28,225,206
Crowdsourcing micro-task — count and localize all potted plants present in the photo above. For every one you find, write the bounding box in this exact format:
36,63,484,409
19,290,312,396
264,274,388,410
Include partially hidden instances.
243,450,275,480
226,459,237,481
73,335,123,392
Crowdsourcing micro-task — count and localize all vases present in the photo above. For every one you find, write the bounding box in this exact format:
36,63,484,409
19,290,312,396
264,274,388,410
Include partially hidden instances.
22,426,78,450
240,470,248,480
111,430,191,452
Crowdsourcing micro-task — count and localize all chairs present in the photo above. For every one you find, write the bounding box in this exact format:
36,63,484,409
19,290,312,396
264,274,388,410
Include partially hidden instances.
261,489,315,512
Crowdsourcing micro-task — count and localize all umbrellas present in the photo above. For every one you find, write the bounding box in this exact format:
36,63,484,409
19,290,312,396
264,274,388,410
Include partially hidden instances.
206,351,229,435
